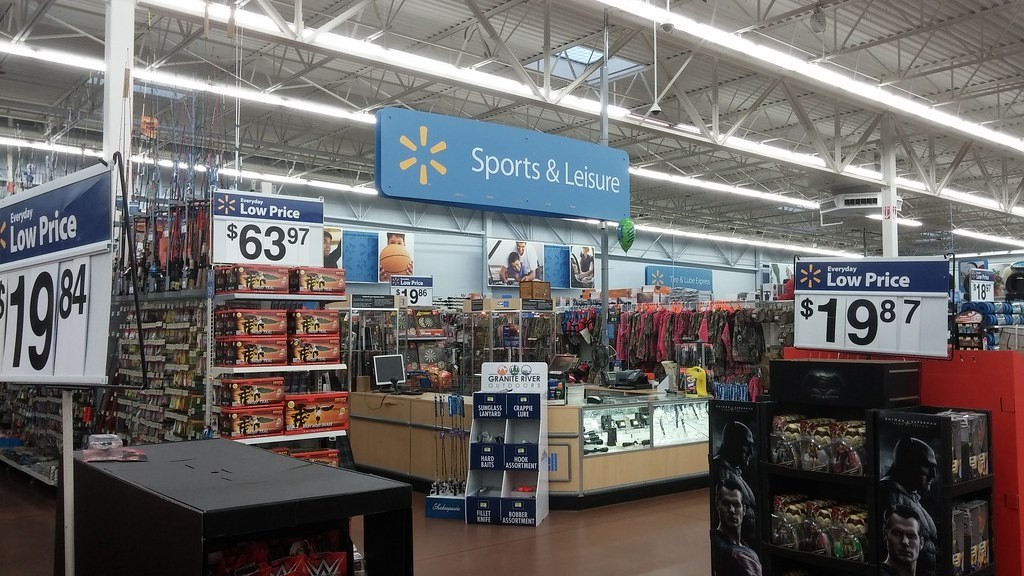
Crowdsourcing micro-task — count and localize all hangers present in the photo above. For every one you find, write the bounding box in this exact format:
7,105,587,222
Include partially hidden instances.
564,299,750,311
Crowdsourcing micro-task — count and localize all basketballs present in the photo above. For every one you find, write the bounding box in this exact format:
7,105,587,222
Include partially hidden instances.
380,244,412,280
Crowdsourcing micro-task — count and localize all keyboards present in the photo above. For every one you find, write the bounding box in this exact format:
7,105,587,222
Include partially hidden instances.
402,390,423,396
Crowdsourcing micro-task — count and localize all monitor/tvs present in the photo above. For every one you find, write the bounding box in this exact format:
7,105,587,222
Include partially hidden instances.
373,354,405,393
548,353,577,375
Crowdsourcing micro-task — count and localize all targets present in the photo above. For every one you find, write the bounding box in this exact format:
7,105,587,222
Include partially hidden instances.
418,317,433,328
423,348,437,364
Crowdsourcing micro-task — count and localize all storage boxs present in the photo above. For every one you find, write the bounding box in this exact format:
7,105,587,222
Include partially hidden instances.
287,338,341,366
519,281,670,311
287,309,340,337
218,408,285,440
216,309,288,339
937,409,990,576
284,389,349,435
220,376,286,410
218,338,287,366
214,265,346,296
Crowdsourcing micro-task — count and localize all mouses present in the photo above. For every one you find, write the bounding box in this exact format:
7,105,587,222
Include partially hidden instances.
414,388,420,392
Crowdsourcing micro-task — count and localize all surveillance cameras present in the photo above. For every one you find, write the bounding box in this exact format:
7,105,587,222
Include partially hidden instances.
660,19,674,32
650,104,662,115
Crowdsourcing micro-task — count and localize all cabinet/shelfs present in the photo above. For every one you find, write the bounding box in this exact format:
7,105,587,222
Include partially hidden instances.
464,361,550,528
761,359,922,576
205,264,347,445
877,404,997,576
53,437,414,576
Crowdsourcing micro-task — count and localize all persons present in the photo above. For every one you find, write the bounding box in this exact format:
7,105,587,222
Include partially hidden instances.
711,421,763,576
499,242,535,285
880,437,939,576
324,230,341,268
380,233,413,282
579,247,594,275
